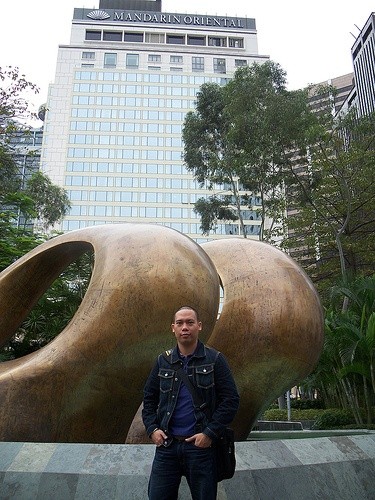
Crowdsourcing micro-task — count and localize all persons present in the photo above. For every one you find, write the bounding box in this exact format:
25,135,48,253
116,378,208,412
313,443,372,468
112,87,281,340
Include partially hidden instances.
141,305,240,500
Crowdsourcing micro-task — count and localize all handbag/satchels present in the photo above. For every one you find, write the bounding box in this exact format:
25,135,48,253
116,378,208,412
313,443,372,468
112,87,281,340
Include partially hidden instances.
215,428,236,483
